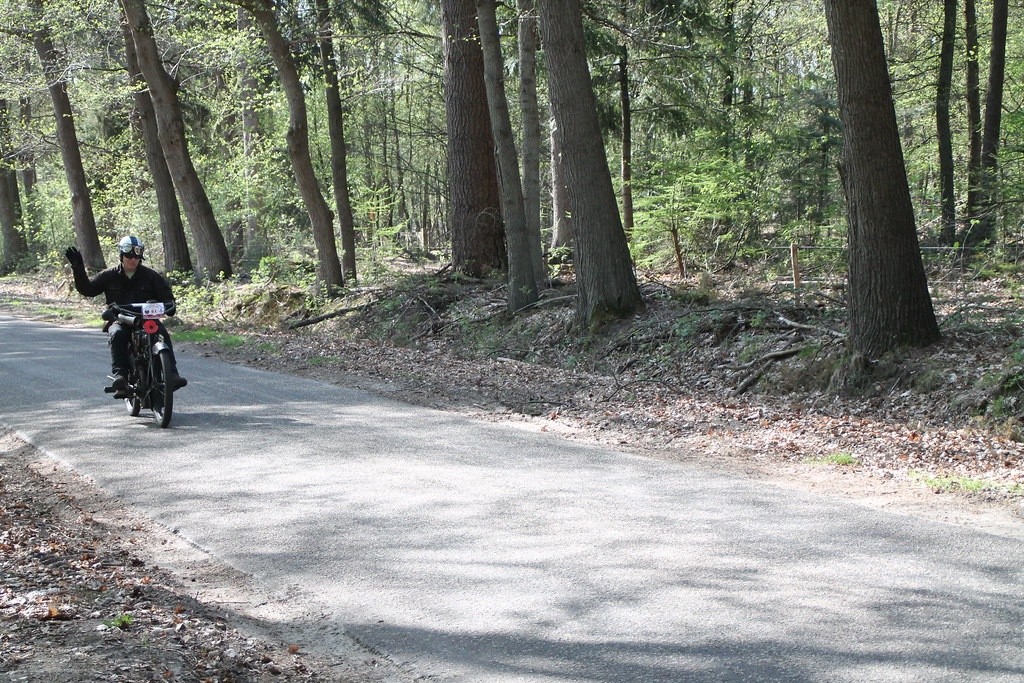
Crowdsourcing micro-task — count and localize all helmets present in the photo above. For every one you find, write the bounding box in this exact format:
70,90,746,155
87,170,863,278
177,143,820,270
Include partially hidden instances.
119,236,145,261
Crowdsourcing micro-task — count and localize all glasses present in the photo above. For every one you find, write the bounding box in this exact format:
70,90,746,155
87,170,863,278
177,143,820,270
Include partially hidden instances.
120,243,143,256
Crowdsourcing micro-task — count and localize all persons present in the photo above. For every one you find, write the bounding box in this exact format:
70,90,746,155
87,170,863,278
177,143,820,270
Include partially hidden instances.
66,236,187,390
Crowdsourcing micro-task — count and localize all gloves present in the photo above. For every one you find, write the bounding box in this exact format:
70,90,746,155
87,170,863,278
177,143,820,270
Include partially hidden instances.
65,246,83,266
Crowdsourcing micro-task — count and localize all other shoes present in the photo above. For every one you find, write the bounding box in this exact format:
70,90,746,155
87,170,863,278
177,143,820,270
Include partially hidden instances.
112,375,127,388
172,372,187,391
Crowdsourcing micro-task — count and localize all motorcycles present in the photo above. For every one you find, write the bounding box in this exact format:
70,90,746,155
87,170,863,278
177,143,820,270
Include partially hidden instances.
101,299,184,429
